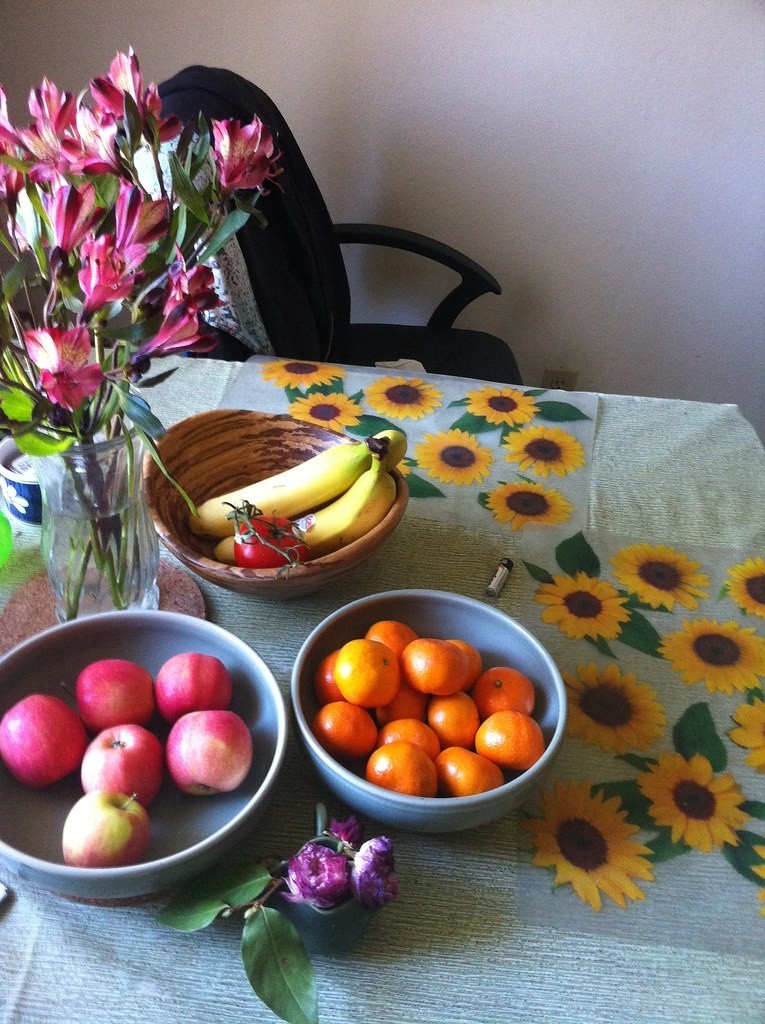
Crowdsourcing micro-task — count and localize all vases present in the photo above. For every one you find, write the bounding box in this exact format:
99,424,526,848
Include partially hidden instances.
36,426,158,624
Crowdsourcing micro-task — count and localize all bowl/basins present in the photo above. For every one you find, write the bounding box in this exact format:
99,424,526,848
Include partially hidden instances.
0,610,289,898
0,428,48,525
291,589,567,833
143,408,408,601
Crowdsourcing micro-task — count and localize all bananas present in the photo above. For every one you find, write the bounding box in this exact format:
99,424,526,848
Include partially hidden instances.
190,430,407,563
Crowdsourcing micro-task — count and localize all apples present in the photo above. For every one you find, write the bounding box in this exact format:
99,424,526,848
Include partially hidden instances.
0,652,252,867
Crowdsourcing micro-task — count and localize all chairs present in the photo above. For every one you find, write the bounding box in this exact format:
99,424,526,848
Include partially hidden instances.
150,64,525,385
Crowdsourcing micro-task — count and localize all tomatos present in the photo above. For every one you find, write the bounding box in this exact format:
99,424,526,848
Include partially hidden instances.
235,516,306,569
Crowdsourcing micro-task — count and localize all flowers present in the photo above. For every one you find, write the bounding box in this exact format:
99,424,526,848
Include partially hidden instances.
154,817,395,1024
0,45,285,622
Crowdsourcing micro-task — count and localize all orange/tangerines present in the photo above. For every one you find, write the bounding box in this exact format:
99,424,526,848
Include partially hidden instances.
313,620,545,797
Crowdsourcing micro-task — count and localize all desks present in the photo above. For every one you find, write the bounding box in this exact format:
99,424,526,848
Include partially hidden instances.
0,344,763,1024
